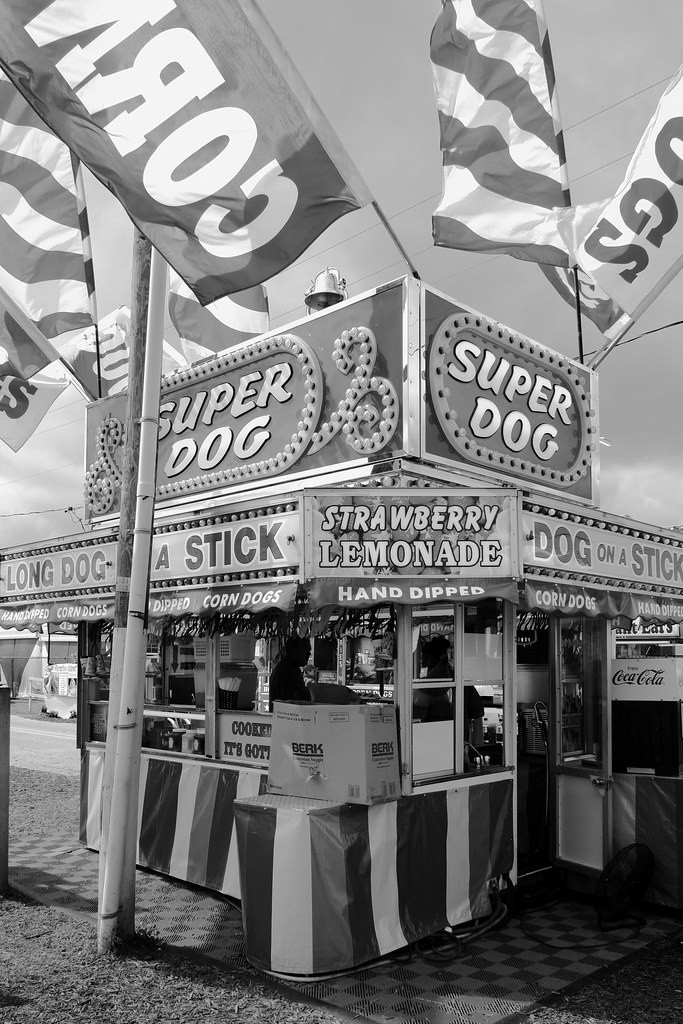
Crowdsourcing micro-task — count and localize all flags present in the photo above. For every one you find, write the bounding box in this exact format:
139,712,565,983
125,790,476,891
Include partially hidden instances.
429,0,577,269
0,0,375,453
537,63,683,342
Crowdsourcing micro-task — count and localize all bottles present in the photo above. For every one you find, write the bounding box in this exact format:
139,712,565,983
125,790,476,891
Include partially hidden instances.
167,727,205,755
482,718,489,743
496,719,503,743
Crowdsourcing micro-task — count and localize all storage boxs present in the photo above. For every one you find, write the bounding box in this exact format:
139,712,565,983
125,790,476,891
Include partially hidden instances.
266,699,402,805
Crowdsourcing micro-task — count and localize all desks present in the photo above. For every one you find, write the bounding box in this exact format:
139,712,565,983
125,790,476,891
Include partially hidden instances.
233,794,402,974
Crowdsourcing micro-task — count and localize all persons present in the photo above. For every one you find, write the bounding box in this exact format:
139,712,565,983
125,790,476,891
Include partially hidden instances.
412,635,484,750
269,635,311,714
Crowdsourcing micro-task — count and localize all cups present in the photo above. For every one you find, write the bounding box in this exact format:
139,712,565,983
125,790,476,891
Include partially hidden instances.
80,658,88,679
85,656,95,677
96,654,106,674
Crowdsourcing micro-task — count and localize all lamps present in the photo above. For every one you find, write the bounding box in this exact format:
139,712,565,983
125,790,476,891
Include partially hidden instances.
305,267,347,316
